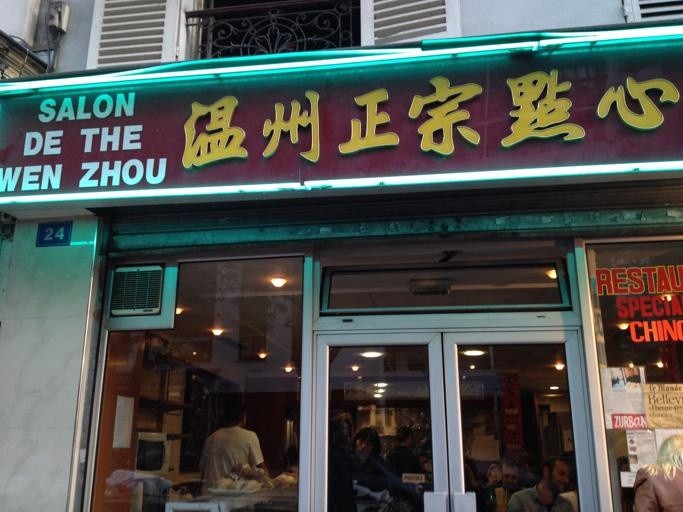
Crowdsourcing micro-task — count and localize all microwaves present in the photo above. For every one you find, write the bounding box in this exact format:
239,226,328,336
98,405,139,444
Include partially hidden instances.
132,433,172,475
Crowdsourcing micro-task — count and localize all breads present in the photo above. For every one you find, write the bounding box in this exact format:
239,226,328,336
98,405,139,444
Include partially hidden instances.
214,464,298,491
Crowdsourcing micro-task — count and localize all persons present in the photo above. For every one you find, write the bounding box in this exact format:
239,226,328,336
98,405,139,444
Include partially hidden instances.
278,414,522,512
631,432,682,511
506,456,573,512
199,404,269,499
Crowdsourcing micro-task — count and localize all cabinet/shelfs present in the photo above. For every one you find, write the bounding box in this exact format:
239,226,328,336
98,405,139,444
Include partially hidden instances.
138,350,184,411
180,363,239,474
